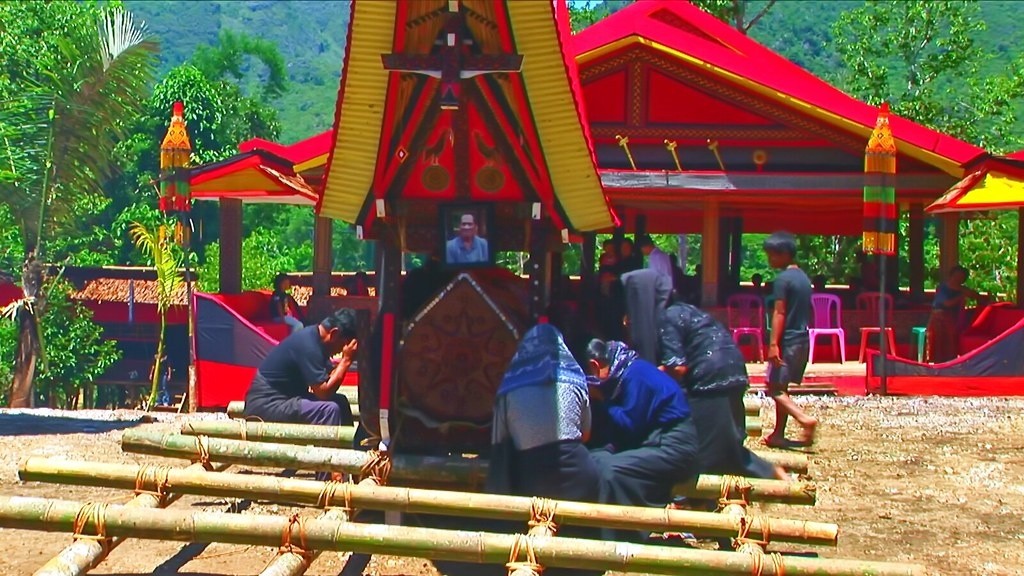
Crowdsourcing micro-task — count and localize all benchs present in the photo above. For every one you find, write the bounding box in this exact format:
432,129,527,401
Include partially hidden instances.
192,290,303,409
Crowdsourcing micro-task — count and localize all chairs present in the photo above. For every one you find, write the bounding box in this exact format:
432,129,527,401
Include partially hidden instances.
724,291,896,365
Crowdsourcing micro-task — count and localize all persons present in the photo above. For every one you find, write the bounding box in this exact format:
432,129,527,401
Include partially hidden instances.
447,214,489,263
491,235,866,543
269,273,304,335
245,308,360,481
337,271,369,297
149,354,171,406
925,265,979,365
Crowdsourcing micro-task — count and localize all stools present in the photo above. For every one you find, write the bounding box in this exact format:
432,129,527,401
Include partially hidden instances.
913,326,926,362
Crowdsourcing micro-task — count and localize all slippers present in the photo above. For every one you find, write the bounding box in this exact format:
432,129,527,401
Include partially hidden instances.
758,434,785,448
